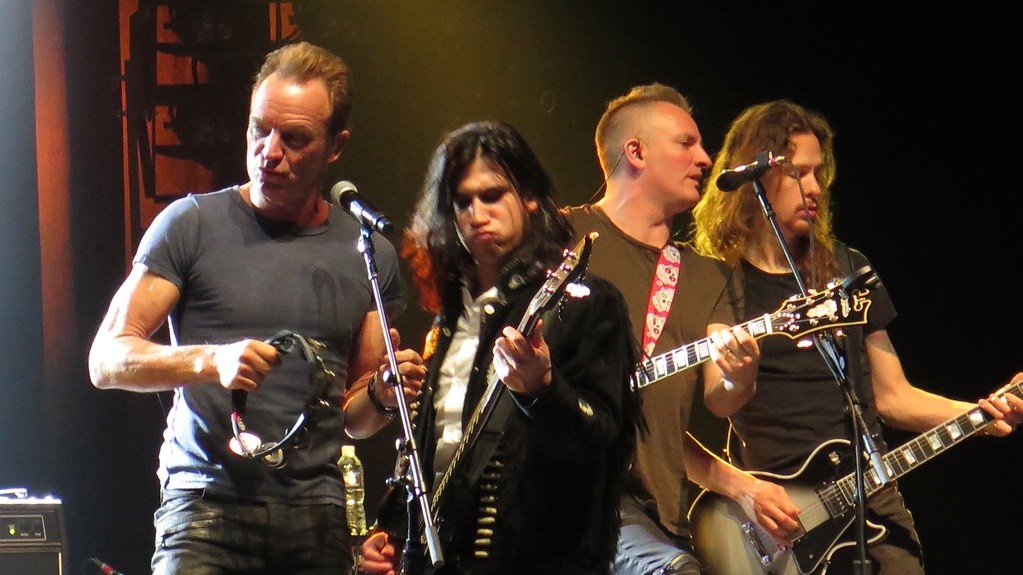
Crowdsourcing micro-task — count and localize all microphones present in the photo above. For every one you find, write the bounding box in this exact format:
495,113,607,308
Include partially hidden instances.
716,156,787,194
92,558,123,575
330,182,395,237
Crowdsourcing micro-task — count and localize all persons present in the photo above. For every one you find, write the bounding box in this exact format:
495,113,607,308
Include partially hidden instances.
357,120,650,575
683,99,1022,575
562,81,759,575
89,41,425,575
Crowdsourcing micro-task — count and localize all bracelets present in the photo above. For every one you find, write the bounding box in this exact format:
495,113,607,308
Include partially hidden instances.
367,373,398,419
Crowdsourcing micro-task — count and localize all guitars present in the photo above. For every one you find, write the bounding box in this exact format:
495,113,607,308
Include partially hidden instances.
630,265,879,390
398,230,599,575
689,377,1022,574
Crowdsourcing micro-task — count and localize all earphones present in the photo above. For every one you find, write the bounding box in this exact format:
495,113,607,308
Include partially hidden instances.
632,149,642,160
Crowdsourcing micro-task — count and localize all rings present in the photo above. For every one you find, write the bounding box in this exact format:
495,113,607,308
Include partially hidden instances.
723,349,731,360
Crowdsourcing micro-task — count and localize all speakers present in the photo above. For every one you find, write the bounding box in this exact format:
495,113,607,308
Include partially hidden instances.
0,496,72,575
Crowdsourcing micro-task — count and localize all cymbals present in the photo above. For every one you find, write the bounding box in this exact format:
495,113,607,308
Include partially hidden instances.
229,329,335,469
